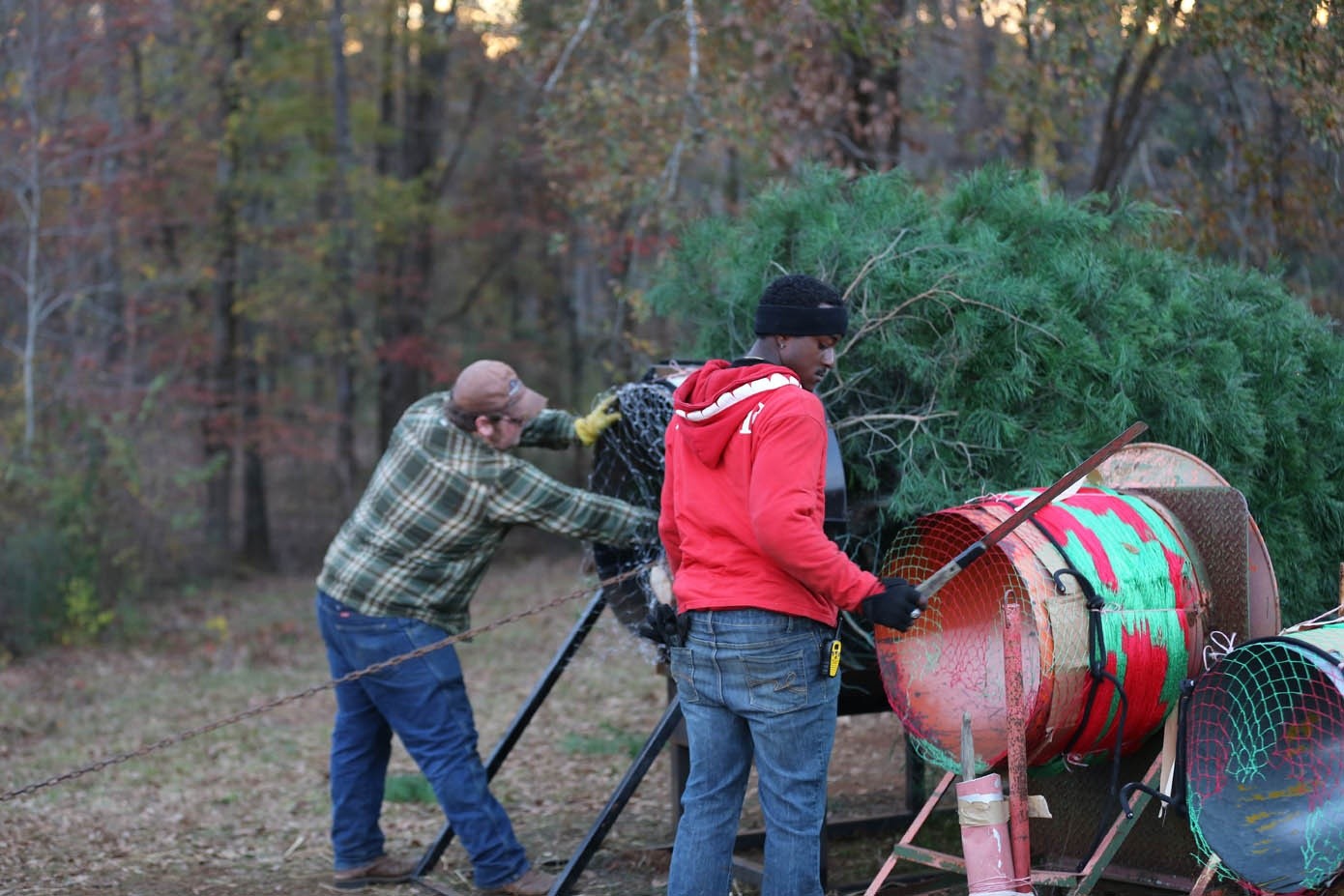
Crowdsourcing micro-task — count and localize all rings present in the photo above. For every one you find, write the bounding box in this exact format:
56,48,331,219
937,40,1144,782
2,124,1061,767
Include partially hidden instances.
910,608,921,618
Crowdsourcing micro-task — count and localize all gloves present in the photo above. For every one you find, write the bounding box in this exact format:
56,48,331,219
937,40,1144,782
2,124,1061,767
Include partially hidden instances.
575,394,621,447
641,604,690,647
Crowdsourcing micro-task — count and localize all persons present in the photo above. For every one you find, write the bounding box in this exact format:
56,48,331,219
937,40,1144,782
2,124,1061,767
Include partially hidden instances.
658,275,926,896
316,360,659,896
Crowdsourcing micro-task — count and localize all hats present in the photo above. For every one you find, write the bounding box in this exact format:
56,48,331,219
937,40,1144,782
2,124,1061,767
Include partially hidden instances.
454,359,548,420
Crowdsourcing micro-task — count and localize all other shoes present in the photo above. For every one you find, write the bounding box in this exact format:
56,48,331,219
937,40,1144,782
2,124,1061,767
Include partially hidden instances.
333,855,420,889
479,867,558,896
